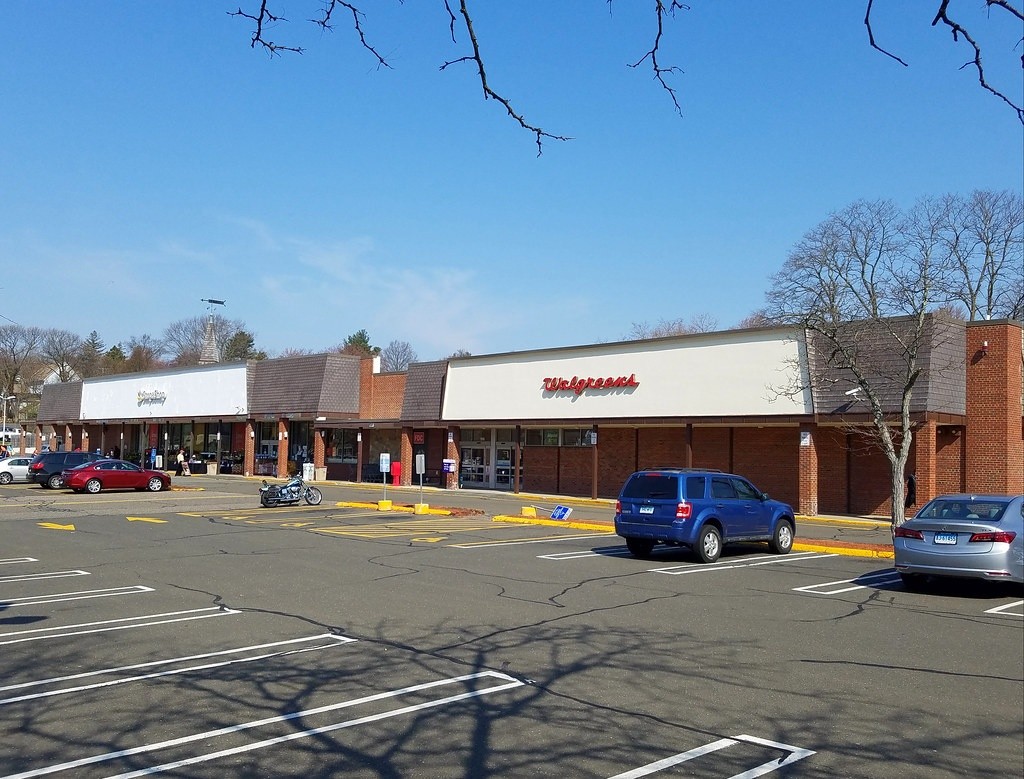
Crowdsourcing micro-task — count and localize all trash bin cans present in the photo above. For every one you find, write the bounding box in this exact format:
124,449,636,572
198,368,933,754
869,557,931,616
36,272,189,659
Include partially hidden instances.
315,466,328,482
206,461,218,476
302,463,315,482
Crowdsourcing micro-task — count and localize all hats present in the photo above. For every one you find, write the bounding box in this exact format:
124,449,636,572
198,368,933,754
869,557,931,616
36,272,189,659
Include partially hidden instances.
179,449,184,453
192,455,196,458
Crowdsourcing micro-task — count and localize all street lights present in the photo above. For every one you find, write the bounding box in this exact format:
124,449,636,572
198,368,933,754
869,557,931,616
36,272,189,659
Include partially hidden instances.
0,395,16,446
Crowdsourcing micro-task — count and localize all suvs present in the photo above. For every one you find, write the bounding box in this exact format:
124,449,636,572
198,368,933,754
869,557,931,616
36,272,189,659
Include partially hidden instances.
26,449,116,491
614,466,798,564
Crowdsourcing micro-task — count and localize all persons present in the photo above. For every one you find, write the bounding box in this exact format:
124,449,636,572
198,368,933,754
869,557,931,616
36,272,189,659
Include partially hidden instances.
105,445,121,460
0,443,10,458
189,455,198,464
262,478,270,488
175,450,185,475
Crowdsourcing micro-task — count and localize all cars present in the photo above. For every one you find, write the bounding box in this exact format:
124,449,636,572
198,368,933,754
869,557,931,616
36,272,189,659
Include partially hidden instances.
1,455,36,486
894,493,1024,590
59,459,172,494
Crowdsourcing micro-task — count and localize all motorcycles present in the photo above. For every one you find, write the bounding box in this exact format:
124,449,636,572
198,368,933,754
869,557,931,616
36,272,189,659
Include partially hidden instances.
256,471,323,511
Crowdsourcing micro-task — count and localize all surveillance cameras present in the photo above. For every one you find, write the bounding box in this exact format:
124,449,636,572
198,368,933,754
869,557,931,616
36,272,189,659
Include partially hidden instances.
845,387,862,395
